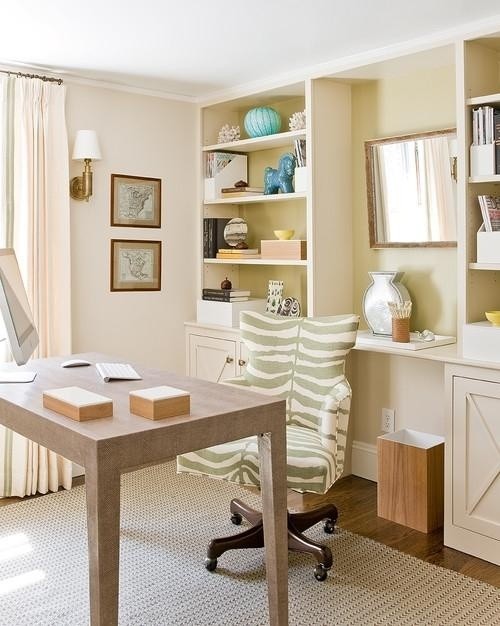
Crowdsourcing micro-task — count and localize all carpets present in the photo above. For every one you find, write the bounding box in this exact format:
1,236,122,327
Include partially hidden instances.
0,457,500,626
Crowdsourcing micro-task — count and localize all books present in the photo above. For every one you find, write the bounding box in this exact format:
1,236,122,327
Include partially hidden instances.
205,217,260,259
467,106,500,233
205,151,266,199
204,287,250,302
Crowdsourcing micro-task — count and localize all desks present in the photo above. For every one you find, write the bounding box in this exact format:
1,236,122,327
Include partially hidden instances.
0,352,288,626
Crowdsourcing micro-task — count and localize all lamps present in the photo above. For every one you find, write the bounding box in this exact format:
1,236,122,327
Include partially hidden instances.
70,129,103,200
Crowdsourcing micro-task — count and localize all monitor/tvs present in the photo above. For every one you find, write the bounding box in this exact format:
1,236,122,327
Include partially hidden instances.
0,247,39,383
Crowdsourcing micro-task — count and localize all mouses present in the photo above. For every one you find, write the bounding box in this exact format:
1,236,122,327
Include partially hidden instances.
61,359,91,368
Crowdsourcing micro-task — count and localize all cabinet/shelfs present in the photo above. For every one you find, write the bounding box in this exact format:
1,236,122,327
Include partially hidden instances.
444,363,500,568
183,12,500,567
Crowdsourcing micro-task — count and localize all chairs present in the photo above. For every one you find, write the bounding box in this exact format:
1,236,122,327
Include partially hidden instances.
175,312,360,582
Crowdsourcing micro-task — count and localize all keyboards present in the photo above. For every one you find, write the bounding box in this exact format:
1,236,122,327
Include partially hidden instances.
96,363,143,383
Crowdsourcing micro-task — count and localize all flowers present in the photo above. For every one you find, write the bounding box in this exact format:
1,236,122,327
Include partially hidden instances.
217,123,241,142
289,109,305,130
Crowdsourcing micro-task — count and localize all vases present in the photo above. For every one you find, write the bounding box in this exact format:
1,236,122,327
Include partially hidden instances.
361,271,411,334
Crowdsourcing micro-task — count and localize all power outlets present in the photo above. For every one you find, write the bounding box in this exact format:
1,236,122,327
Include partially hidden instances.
380,408,395,434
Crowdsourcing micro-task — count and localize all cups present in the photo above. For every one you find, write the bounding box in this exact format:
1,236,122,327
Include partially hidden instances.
391,317,410,343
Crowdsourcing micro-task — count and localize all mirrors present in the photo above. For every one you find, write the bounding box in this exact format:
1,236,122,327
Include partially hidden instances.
364,127,459,248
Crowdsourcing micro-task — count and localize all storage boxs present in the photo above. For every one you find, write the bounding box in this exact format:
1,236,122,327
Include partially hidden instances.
462,324,500,364
196,296,268,330
260,241,306,261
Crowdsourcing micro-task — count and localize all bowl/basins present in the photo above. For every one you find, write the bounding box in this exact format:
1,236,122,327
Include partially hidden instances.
272,231,295,240
484,310,500,326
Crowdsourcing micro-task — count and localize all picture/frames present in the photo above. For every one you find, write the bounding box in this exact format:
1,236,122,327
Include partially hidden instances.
111,174,161,228
111,239,160,292
